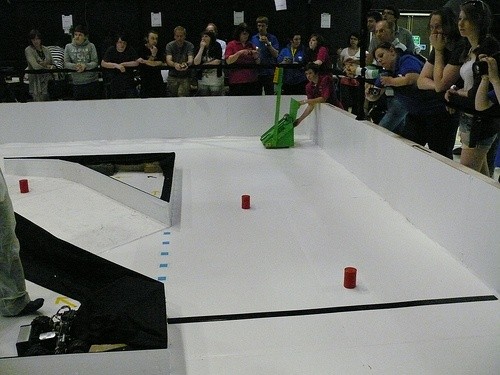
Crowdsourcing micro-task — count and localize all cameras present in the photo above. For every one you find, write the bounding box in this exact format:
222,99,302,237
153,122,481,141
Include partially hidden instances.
472,37,500,75
369,88,381,95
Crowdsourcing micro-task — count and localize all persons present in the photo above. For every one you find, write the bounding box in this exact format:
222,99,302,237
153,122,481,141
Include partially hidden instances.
250,16,279,96
417,7,470,157
0,169,44,319
364,11,383,66
64,25,98,100
307,33,332,70
334,39,348,68
224,23,261,95
375,20,414,133
381,6,414,52
474,54,500,183
194,32,223,96
277,31,307,95
206,23,226,60
293,63,344,127
24,28,56,101
445,0,500,175
140,31,166,98
166,26,194,97
338,33,370,113
373,43,440,157
101,33,139,98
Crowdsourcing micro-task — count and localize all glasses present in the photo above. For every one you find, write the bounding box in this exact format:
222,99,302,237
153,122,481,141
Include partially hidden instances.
428,22,442,31
382,12,395,16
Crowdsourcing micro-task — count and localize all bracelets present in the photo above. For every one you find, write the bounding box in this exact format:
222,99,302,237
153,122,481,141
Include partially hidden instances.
265,41,271,46
